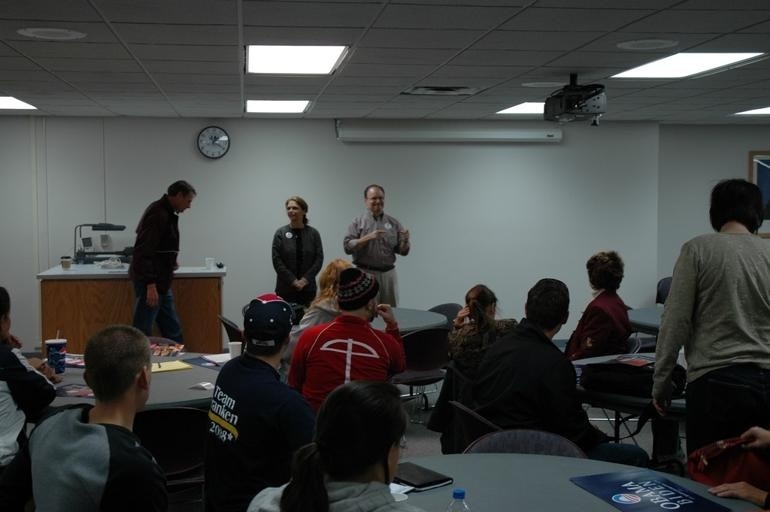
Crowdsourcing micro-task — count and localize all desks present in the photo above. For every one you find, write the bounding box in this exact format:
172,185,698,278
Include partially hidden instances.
570,275,687,470
370,308,448,414
43,347,217,483
265,451,761,512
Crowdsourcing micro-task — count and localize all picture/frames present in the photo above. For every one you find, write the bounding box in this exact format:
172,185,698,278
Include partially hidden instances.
748,150,770,238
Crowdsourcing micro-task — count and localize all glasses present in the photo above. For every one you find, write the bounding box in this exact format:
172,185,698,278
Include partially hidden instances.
367,197,386,201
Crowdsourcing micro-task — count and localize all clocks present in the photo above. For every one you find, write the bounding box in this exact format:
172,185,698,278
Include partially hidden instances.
197,127,231,159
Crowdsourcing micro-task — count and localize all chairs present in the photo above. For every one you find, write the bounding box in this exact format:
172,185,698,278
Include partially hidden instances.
386,303,468,425
461,430,581,456
215,313,243,343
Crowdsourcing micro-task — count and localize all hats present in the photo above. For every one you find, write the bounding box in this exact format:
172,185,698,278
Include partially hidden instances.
338,268,380,312
243,294,296,347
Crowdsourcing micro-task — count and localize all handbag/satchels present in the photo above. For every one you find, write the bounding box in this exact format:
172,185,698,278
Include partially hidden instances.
689,437,770,488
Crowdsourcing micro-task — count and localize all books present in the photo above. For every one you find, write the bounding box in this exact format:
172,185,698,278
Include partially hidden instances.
393,462,453,492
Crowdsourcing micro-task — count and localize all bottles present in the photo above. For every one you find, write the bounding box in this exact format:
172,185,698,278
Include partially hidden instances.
445,489,471,512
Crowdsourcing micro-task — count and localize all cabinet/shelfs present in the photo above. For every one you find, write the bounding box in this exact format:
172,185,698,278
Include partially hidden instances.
37,258,226,356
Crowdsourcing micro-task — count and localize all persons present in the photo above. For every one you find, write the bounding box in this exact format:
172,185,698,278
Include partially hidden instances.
0,286,55,506
443,283,516,361
561,250,632,368
653,178,770,458
280,258,357,384
271,197,324,309
343,184,412,308
284,268,406,412
428,280,650,467
708,425,770,511
252,381,425,512
203,294,317,512
129,180,197,347
30,324,186,512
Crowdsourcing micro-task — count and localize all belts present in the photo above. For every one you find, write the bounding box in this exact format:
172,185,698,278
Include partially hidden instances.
362,266,394,273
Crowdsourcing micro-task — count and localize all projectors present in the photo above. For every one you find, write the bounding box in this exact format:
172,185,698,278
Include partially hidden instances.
543,72,607,127
